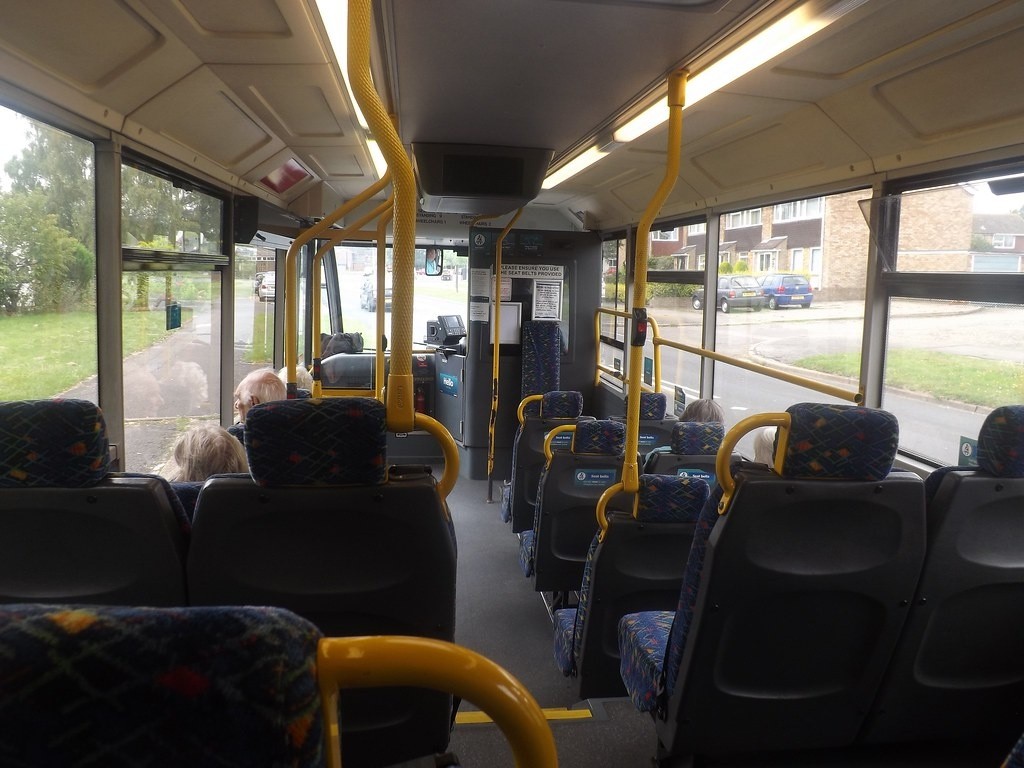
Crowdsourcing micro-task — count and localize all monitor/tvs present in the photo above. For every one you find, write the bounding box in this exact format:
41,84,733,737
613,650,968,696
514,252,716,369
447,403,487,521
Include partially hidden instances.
442,153,524,196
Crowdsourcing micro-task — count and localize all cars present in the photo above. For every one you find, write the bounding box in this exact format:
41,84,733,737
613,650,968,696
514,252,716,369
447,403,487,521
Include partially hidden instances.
361,264,394,313
441,269,451,281
757,273,813,309
254,270,276,302
691,273,766,312
416,270,426,275
605,265,616,275
321,266,327,289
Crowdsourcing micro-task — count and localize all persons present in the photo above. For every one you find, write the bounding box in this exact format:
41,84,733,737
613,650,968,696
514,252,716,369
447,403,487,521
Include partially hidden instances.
679,398,725,422
172,424,247,482
235,370,288,423
279,365,313,390
754,425,779,467
426,249,438,274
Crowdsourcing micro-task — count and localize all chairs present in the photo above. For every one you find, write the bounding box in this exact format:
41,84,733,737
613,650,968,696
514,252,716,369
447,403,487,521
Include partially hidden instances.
2,389,1023,768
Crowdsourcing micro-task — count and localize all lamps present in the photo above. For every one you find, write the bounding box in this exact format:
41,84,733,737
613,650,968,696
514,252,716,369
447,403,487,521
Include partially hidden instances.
309,1,860,183
